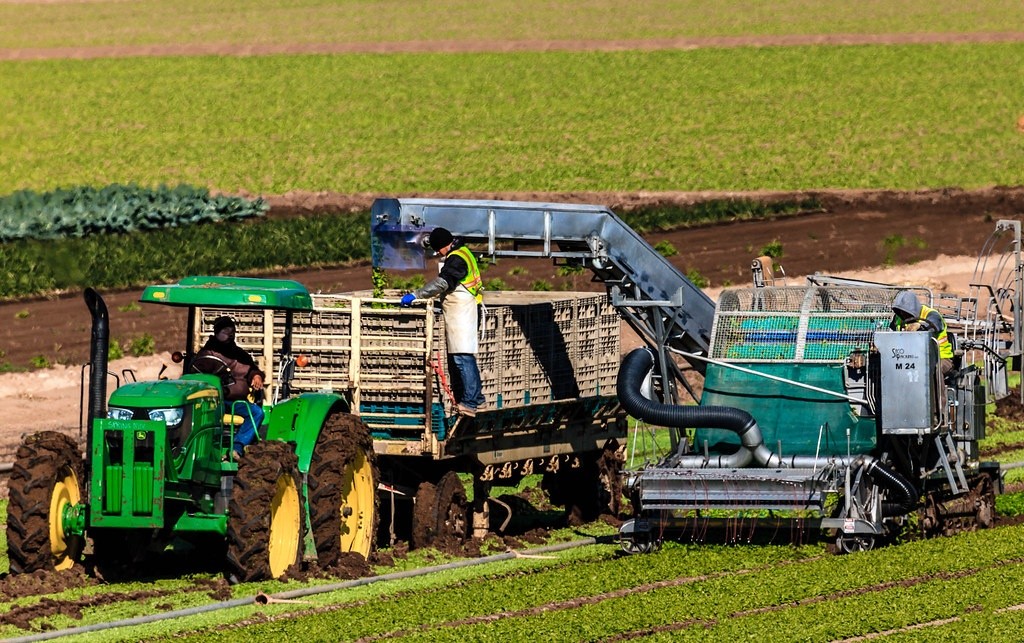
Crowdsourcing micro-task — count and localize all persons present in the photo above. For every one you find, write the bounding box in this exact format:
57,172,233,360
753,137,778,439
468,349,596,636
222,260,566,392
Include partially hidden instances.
401,227,487,409
188,316,265,462
889,291,954,425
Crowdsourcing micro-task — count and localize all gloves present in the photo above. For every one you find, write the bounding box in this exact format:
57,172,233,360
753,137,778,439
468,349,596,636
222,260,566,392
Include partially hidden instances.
401,292,417,307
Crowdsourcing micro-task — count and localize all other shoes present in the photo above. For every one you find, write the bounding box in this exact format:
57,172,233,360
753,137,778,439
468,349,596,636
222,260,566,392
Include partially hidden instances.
444,401,477,418
476,401,489,412
227,451,241,463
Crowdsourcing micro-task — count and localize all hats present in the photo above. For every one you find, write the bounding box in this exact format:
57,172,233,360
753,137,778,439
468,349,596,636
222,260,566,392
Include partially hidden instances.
429,227,453,252
214,316,236,335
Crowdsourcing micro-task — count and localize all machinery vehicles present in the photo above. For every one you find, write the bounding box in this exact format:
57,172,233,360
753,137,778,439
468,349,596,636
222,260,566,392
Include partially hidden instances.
6,283,380,581
367,198,1022,556
